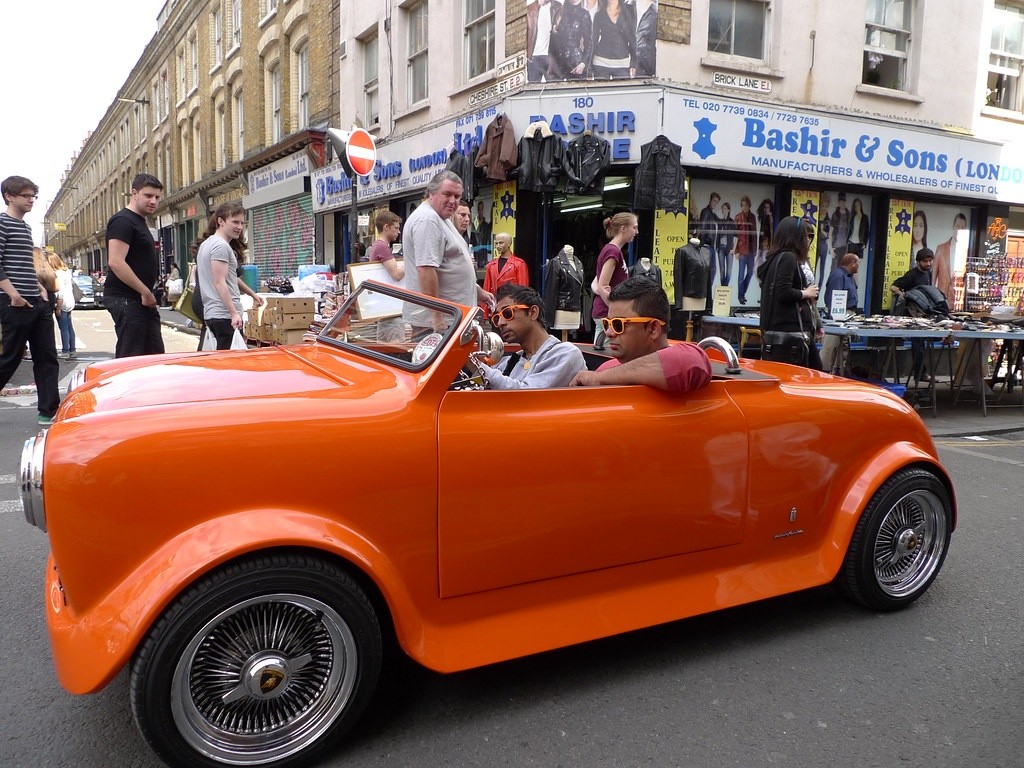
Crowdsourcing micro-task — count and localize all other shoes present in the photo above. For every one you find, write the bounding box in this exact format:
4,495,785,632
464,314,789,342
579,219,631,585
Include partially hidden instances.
38,416,54,424
739,296,747,304
21,353,32,361
915,375,938,383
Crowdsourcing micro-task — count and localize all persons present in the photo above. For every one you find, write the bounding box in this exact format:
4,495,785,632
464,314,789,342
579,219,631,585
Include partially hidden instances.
815,193,869,292
191,204,264,351
462,201,495,266
402,171,496,343
104,173,180,357
674,238,712,311
432,283,588,391
757,217,825,371
481,233,529,335
628,258,663,287
890,248,939,383
527,0,658,81
568,278,713,394
369,211,407,343
820,254,860,375
0,175,76,425
910,212,968,308
689,192,778,305
591,213,639,347
544,244,584,330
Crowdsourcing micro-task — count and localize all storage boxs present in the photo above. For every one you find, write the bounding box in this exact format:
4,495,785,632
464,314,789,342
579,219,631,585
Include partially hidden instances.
860,379,906,397
245,297,315,345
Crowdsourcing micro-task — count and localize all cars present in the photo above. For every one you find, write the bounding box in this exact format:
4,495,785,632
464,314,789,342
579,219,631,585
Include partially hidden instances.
72,276,104,310
19,277,958,768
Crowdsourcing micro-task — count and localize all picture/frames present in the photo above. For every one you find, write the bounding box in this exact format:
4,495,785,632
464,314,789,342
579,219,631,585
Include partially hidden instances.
346,257,405,322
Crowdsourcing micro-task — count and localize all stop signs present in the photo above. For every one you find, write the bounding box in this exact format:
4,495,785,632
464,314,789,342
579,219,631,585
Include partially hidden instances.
346,127,376,176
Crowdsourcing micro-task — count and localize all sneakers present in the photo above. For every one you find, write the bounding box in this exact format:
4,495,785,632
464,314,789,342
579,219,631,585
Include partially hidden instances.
57,351,70,359
69,351,77,358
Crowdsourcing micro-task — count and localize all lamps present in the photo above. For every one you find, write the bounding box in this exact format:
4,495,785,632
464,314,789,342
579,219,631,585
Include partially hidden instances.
118,96,149,107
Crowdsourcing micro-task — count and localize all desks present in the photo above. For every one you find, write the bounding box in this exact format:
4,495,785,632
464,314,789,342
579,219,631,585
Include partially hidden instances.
703,315,1024,418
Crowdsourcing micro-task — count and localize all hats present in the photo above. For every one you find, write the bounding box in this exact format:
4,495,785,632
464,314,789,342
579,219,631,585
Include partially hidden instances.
916,249,934,262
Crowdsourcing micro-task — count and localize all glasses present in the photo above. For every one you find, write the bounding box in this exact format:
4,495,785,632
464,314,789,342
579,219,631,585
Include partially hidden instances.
490,305,530,327
601,317,666,334
18,194,38,201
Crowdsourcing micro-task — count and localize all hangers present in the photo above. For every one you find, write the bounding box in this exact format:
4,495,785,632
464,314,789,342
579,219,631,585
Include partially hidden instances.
451,106,666,159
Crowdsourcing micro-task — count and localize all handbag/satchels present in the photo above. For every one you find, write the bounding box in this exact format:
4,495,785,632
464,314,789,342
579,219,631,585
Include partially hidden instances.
201,325,217,351
71,280,83,302
761,330,810,367
169,279,183,295
230,328,248,350
174,286,203,324
